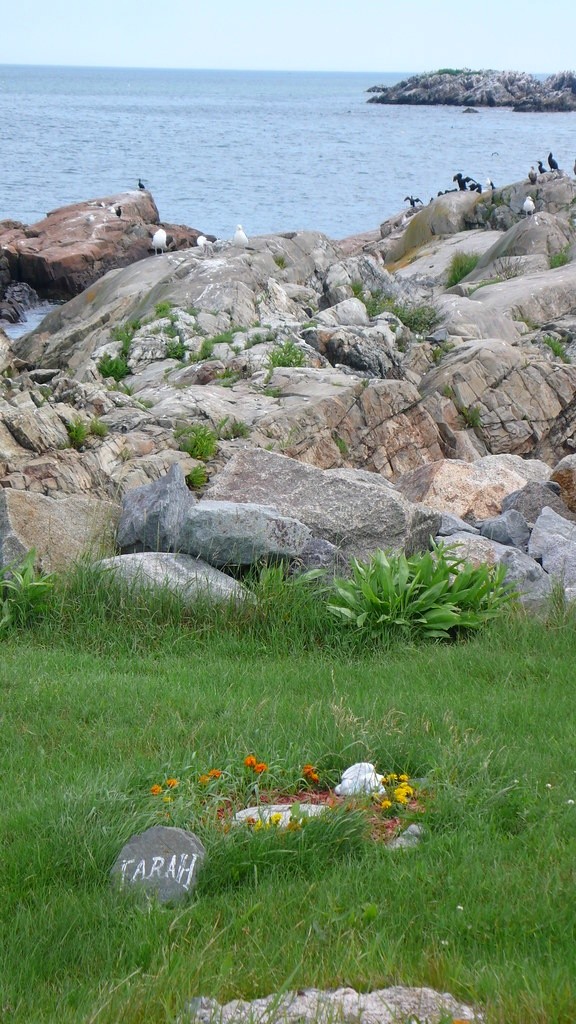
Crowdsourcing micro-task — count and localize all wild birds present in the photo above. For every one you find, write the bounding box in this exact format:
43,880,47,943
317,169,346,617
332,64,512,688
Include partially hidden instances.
153,230,166,253
138,179,145,190
404,150,576,221
232,223,250,252
115,205,123,218
